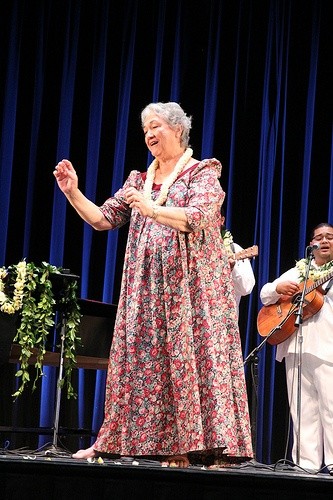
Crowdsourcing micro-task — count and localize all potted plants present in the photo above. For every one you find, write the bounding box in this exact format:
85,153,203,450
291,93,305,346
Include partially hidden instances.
0,257,80,400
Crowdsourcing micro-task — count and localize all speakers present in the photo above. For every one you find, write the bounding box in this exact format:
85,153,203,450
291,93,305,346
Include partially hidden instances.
52,298,118,358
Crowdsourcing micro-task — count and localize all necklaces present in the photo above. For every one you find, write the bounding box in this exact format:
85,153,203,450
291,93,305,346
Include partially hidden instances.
295,259,333,282
143,147,194,206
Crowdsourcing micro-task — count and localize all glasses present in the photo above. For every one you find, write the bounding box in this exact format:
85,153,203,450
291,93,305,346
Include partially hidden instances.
311,233,333,241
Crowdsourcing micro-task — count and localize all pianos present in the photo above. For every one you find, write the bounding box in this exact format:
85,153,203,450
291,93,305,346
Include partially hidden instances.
0,265,119,450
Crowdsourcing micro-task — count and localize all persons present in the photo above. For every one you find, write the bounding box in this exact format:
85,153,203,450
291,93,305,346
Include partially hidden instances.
218,209,257,326
51,101,255,469
258,223,333,475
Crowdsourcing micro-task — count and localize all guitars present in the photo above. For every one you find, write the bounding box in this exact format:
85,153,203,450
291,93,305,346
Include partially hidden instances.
257,270,333,346
228,244,258,266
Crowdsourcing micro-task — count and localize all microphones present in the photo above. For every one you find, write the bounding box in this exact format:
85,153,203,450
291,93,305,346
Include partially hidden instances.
306,242,320,256
